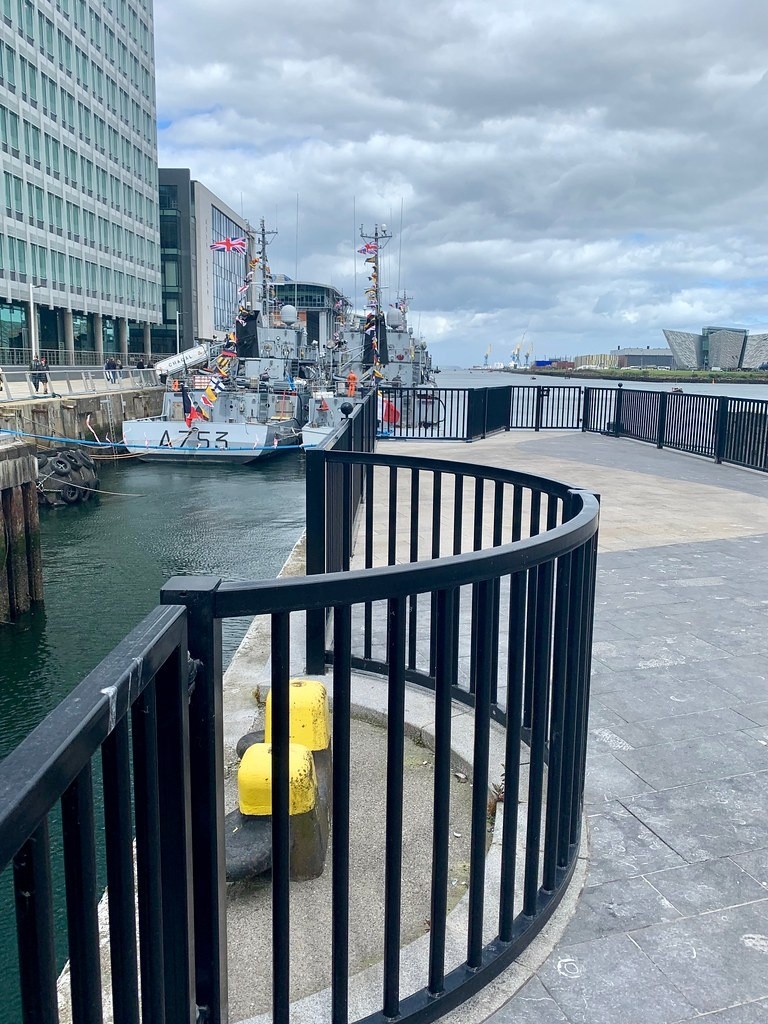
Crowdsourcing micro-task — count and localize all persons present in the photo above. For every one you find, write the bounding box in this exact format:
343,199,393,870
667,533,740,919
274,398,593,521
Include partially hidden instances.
38,357,50,394
116,359,122,380
28,355,41,394
107,357,116,383
147,361,153,368
105,359,109,366
137,358,145,369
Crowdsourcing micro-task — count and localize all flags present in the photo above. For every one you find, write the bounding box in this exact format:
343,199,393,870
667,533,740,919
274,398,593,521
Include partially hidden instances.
210,237,248,256
184,253,413,430
357,242,379,254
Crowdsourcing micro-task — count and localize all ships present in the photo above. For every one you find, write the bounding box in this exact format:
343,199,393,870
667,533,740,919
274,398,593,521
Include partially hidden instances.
118,213,437,466
